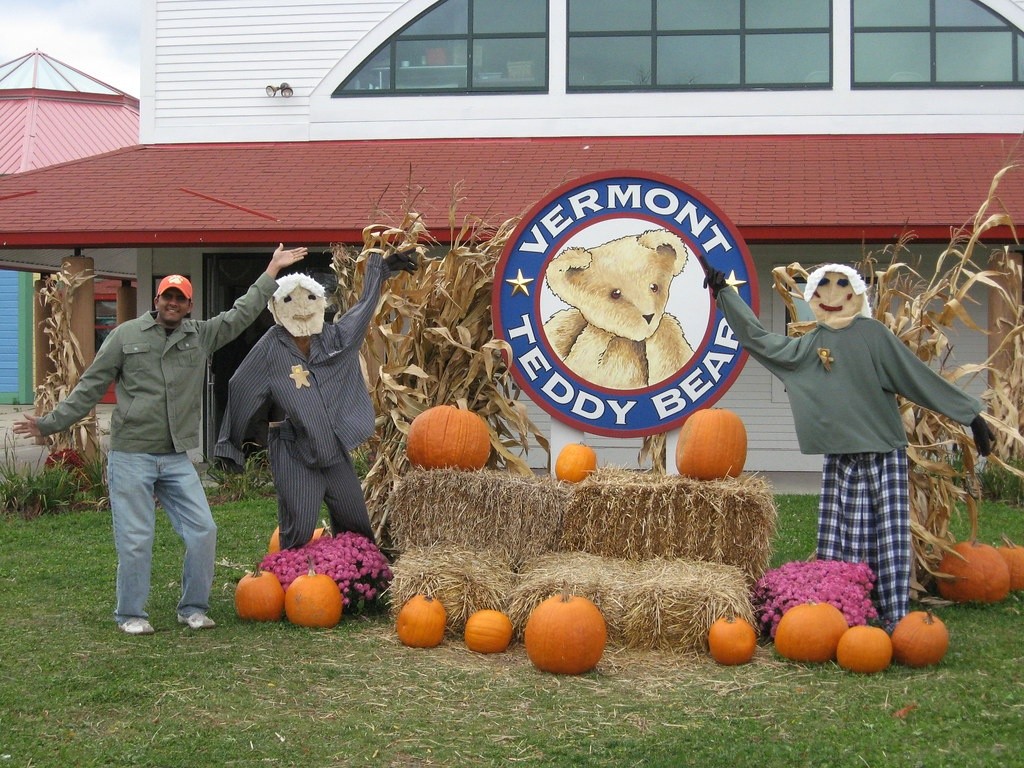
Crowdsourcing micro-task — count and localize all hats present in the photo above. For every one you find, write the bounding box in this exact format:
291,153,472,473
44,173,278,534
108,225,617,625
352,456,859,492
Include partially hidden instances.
157,275,192,301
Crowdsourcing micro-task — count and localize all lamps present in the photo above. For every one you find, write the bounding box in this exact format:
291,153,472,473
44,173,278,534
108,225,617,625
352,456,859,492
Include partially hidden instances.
265,82,293,98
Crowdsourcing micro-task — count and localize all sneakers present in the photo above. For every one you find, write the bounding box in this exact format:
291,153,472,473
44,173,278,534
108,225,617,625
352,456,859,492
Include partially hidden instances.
177,613,215,629
118,617,154,634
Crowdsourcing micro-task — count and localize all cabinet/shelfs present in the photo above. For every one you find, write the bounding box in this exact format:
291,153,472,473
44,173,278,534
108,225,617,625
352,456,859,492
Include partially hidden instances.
371,65,467,88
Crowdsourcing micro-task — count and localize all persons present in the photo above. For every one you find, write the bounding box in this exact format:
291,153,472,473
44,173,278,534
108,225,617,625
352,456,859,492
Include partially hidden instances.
698,254,994,629
214,242,419,616
13,242,308,634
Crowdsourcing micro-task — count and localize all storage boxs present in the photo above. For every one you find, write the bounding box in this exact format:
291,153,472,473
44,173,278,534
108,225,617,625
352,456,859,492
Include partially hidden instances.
452,45,488,67
477,72,504,79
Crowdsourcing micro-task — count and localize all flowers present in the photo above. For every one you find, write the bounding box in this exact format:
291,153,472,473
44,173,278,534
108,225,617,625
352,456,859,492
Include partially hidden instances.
750,559,890,638
260,531,394,618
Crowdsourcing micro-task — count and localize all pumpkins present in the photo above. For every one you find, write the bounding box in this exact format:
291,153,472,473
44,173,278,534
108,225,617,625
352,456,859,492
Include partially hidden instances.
555,441,598,482
406,404,490,470
397,594,447,648
935,538,1011,603
465,610,512,654
708,614,756,665
891,608,948,667
525,586,606,674
993,532,1024,593
675,407,747,480
235,561,284,623
285,555,343,628
775,599,849,661
269,518,329,556
837,625,893,674
